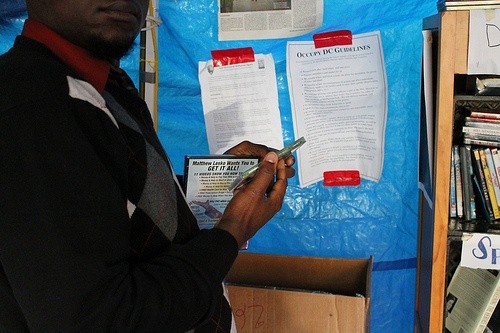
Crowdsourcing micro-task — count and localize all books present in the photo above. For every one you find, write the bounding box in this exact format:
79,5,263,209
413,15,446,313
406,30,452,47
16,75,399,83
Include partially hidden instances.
448,78,500,234
444,260,500,333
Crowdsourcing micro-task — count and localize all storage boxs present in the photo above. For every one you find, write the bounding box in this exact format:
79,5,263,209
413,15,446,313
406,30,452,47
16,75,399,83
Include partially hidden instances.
223,252,373,333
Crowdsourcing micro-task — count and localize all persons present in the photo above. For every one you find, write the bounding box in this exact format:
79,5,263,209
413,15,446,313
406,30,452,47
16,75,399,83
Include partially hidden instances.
0,0,295,333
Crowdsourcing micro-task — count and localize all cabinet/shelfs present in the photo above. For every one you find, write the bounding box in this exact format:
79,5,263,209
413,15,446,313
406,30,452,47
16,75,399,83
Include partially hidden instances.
413,8,500,333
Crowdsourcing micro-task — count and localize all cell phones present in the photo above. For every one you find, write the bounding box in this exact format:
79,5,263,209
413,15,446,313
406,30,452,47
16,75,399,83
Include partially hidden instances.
227,137,306,193
